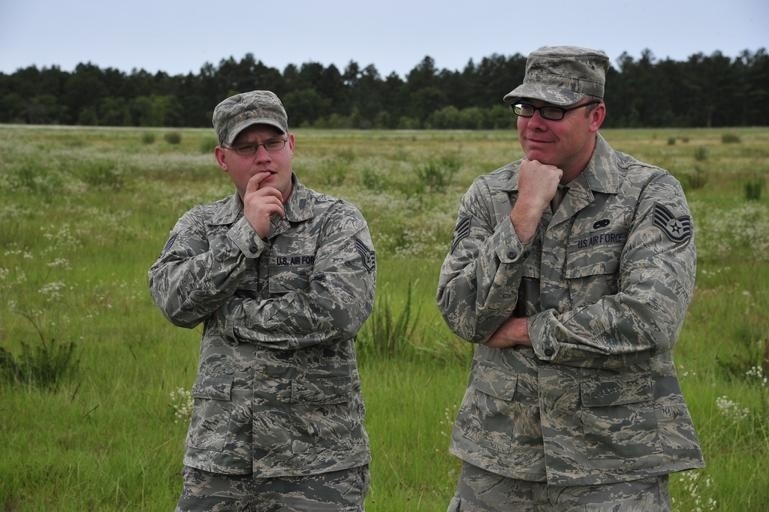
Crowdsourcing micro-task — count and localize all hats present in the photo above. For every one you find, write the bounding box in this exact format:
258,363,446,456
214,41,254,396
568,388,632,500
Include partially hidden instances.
503,45,610,106
212,89,288,144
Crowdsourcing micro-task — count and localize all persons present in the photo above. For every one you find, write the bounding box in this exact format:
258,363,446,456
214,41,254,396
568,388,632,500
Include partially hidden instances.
432,45,710,512
146,89,379,510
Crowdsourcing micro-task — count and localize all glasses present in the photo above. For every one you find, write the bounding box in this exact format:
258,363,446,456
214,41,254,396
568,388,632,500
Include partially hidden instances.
510,99,601,121
222,133,288,156
